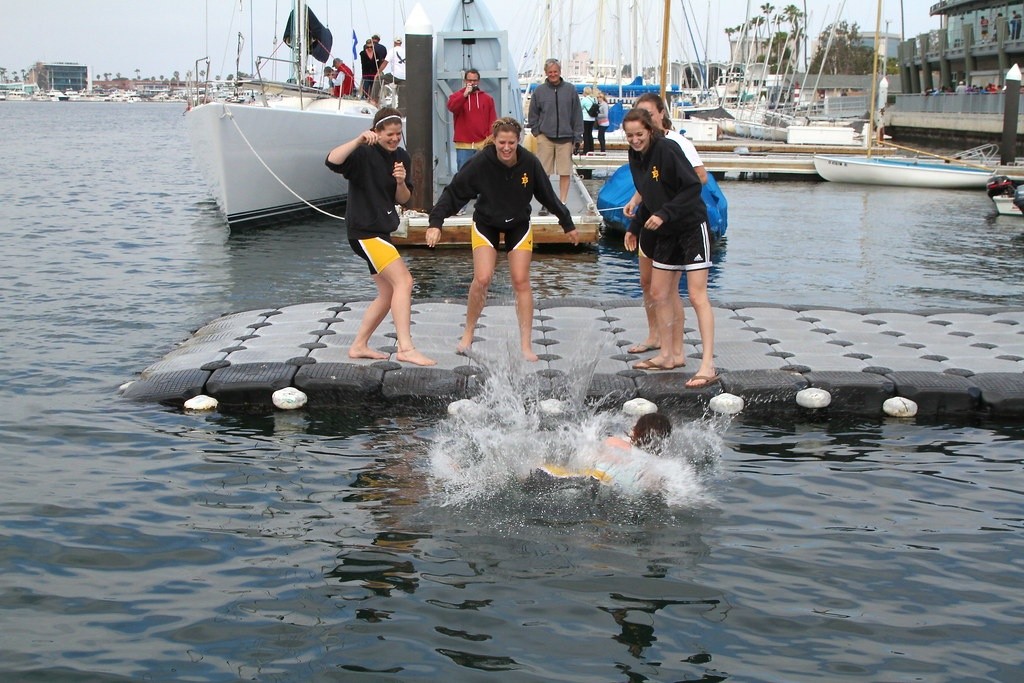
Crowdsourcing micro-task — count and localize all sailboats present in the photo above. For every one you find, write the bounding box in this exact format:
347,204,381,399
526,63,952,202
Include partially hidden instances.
183,0,410,235
510,0,903,146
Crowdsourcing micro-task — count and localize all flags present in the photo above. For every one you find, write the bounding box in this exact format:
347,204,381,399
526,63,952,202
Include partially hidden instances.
353,28,358,61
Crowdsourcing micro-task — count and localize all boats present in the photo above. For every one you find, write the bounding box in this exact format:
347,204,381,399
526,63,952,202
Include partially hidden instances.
0,87,258,104
987,175,1024,216
812,143,1024,190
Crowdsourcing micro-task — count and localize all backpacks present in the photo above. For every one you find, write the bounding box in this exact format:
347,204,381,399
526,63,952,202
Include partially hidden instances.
584,96,600,117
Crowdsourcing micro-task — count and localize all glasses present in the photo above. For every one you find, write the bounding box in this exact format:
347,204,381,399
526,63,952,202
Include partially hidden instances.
366,46,373,48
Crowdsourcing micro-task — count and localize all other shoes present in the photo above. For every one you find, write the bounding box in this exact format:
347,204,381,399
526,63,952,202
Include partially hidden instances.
538,205,549,215
457,206,466,216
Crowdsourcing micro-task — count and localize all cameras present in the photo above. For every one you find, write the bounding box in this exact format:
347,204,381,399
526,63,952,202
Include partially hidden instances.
471,84,479,92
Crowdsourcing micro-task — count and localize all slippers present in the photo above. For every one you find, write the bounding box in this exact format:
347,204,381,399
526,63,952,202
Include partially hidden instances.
673,363,686,370
628,342,661,353
632,356,675,370
685,373,719,388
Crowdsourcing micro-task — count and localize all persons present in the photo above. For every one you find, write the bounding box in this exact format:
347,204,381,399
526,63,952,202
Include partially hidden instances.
323,67,338,95
595,93,609,156
446,68,497,216
325,106,437,367
994,13,1003,29
924,81,967,95
980,16,988,42
624,92,708,368
1011,10,1022,39
328,58,355,97
968,83,1024,93
358,33,387,99
528,58,585,216
425,118,581,362
602,413,671,455
368,35,405,117
624,108,721,389
581,86,598,155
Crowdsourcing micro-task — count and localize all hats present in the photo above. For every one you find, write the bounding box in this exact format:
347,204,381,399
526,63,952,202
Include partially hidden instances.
324,67,331,76
394,37,402,44
372,34,380,41
333,57,340,68
